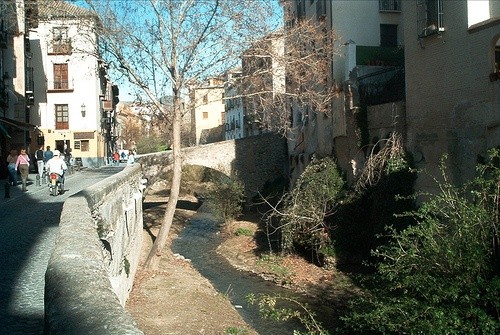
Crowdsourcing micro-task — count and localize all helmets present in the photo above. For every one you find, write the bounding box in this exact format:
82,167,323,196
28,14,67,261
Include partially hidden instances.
53,150,60,156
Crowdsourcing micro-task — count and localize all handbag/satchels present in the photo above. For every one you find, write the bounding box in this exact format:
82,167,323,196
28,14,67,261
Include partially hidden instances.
28,162,29,166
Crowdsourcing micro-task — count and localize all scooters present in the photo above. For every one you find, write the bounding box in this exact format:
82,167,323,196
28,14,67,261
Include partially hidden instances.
49,169,66,196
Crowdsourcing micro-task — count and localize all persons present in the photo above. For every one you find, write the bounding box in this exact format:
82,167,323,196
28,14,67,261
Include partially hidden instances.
34,145,45,185
15,149,31,192
113,150,136,164
45,150,68,194
42,146,53,183
6,149,19,189
